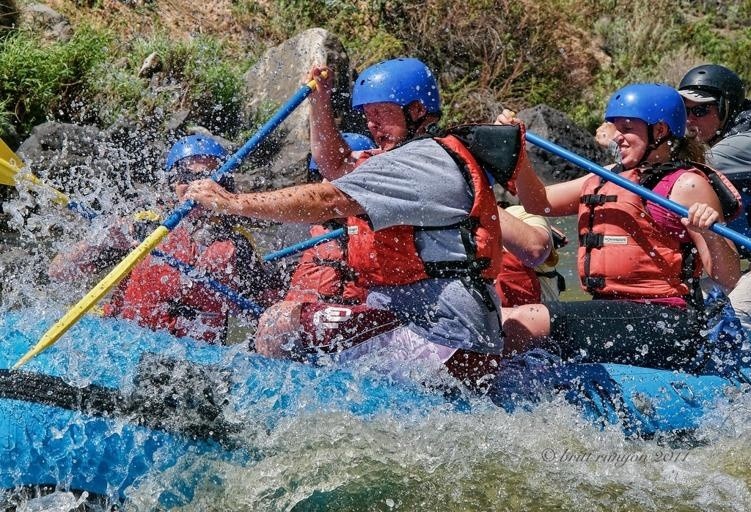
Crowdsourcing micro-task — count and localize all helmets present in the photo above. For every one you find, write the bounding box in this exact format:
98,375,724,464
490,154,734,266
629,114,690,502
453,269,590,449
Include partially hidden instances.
352,59,441,113
308,133,376,170
605,84,686,139
677,64,745,106
165,136,229,170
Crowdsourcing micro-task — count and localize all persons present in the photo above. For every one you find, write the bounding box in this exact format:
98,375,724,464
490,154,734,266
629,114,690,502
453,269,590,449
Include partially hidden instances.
176,56,498,394
500,82,741,367
50,135,287,343
673,64,751,330
473,183,552,308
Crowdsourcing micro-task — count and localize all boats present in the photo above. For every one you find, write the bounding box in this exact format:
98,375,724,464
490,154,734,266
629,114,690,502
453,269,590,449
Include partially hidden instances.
0,306,751,512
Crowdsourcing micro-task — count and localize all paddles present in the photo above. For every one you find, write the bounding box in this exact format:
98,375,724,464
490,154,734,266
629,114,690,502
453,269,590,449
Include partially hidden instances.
0,137,266,318
11,67,329,372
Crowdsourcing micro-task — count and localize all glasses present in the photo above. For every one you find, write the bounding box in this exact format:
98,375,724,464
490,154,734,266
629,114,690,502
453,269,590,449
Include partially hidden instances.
683,105,708,117
